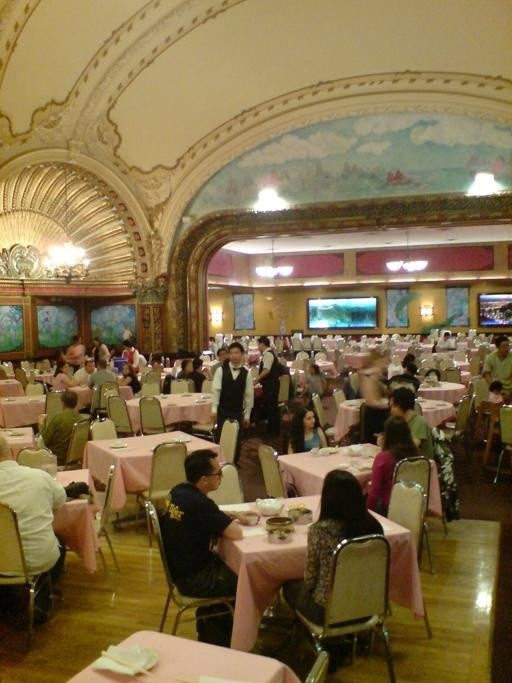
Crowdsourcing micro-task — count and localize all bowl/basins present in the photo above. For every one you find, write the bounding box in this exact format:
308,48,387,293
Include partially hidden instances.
266,517,295,542
256,498,285,515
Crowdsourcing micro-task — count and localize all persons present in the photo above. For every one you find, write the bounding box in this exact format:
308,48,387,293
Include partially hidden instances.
1,439,67,601
308,365,327,398
40,334,143,461
176,335,280,468
368,388,433,517
390,353,421,394
482,336,511,405
162,449,238,599
438,332,450,347
288,408,328,455
281,469,381,672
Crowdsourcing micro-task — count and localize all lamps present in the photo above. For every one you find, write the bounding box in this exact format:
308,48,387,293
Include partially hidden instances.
256,237,295,279
43,162,90,284
385,230,428,272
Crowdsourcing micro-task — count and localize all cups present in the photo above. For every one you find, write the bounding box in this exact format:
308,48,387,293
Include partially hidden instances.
245,514,258,526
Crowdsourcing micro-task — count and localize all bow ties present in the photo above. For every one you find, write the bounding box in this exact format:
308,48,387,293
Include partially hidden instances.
232,366,242,372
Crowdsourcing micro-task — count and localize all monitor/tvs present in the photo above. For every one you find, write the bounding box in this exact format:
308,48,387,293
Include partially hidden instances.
307,295,378,329
478,291,512,327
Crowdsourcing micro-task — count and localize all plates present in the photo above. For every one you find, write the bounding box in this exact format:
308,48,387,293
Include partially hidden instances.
4,368,212,408
8,429,30,438
108,441,128,448
310,378,449,478
132,645,160,671
176,434,193,443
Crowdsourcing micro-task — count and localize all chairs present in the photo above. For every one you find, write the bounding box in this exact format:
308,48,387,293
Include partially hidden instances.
491,405,510,483
144,502,236,635
306,650,330,682
255,444,298,500
393,456,438,574
91,464,122,577
135,440,188,551
0,328,512,472
291,533,396,682
207,463,245,505
385,482,434,641
0,503,53,654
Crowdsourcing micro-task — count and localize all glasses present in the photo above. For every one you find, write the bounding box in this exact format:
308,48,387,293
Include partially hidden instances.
203,469,223,478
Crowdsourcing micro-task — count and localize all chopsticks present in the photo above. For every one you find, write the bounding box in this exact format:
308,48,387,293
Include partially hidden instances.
172,675,200,682
100,651,152,677
